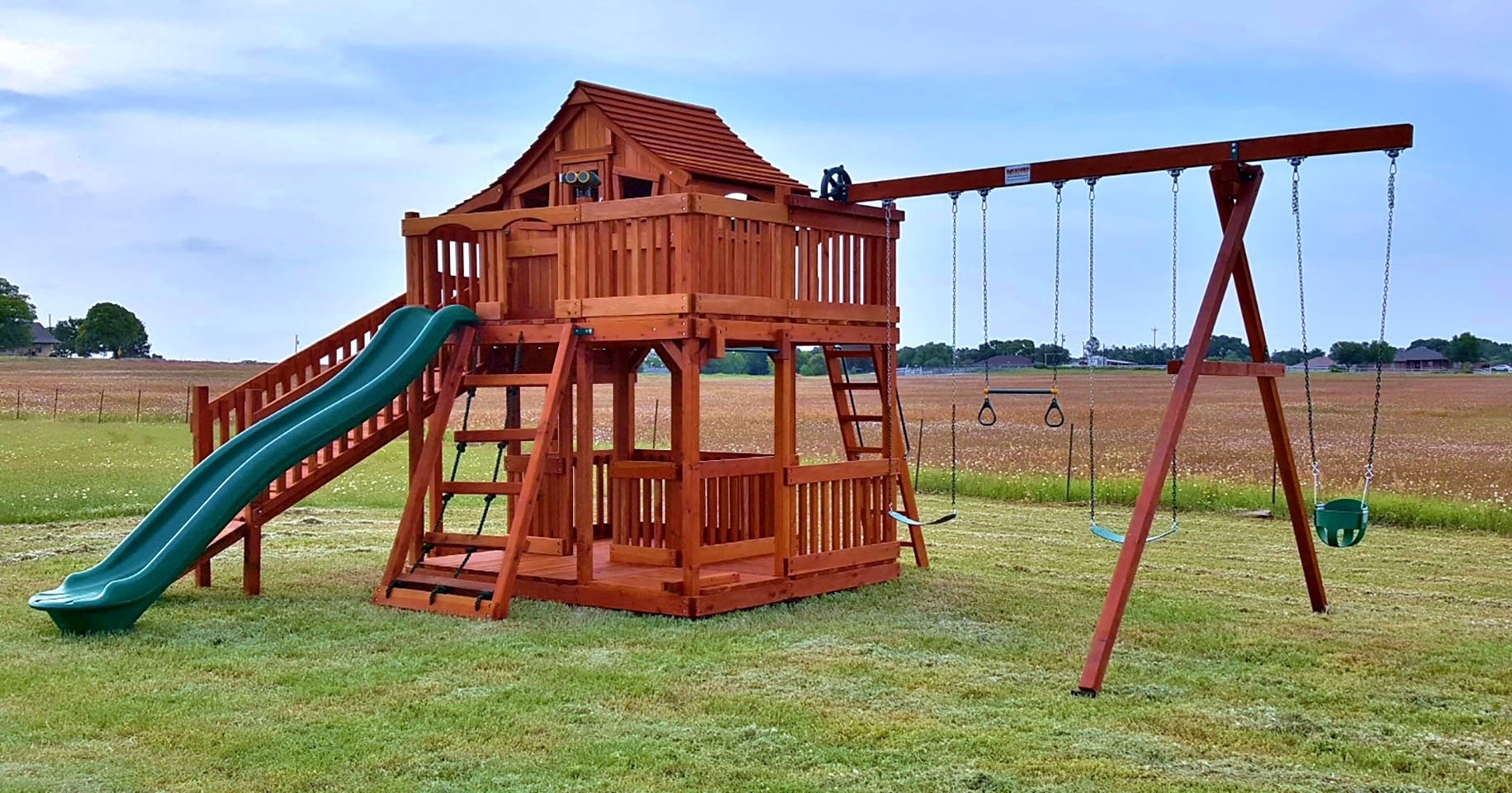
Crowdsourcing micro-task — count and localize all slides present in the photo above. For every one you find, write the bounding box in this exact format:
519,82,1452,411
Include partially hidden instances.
27,302,477,635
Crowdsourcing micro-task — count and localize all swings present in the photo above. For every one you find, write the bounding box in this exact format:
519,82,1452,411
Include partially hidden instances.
1083,168,1186,543
1286,150,1399,550
976,180,1067,427
881,190,963,526
